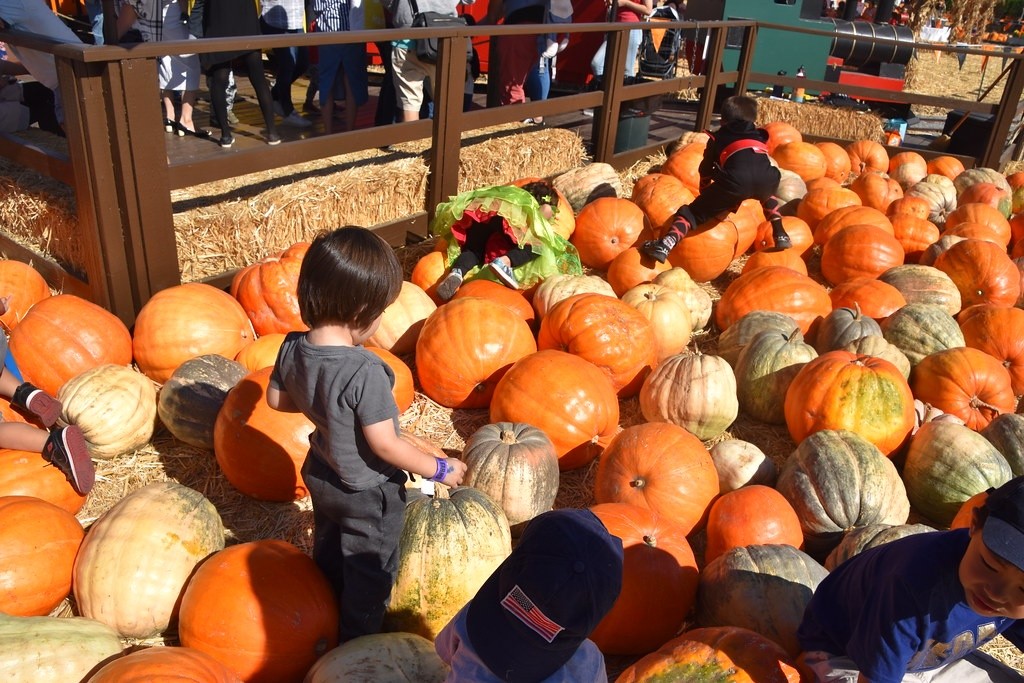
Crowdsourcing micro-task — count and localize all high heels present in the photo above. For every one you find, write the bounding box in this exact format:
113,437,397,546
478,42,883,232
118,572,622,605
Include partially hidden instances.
178,121,213,137
165,117,179,132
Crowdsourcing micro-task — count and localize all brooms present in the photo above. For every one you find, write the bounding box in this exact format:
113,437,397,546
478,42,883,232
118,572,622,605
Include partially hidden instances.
923,59,1016,153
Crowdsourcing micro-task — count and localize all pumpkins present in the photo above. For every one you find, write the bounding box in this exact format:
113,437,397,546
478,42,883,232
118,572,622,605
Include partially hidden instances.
982,32,1008,43
0,122,1024,683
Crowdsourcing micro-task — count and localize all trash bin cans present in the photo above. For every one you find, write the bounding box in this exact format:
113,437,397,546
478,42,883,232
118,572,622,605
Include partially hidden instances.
592,76,654,153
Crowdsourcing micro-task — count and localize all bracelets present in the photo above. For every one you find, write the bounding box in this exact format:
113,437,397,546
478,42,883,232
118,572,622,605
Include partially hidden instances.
427,457,448,482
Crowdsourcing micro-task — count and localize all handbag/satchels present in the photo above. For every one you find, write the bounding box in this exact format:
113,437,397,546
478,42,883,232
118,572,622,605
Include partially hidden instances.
411,11,473,64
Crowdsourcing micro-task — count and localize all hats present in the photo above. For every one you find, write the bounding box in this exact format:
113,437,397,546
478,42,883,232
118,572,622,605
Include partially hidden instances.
982,474,1024,570
466,507,623,682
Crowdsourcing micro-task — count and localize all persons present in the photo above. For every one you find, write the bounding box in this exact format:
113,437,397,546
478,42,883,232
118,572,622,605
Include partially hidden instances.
797,475,1024,683
820,0,946,27
0,0,684,149
434,507,624,683
266,225,467,643
0,295,95,495
428,182,582,300
641,96,792,264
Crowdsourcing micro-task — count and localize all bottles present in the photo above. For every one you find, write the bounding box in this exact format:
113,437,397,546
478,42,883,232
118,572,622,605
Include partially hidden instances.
791,66,806,104
772,70,786,97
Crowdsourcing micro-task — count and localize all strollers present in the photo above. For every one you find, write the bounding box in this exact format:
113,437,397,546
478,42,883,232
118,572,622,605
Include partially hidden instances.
636,5,684,80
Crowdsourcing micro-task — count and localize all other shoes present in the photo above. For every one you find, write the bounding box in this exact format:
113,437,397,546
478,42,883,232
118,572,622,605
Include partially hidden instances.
209,116,229,127
226,111,239,124
435,268,464,300
643,238,671,264
302,103,321,115
773,233,793,250
581,108,594,116
42,425,95,495
282,109,312,127
268,133,285,145
11,381,63,429
332,102,346,111
271,101,285,117
520,115,545,128
488,257,518,289
220,136,235,148
